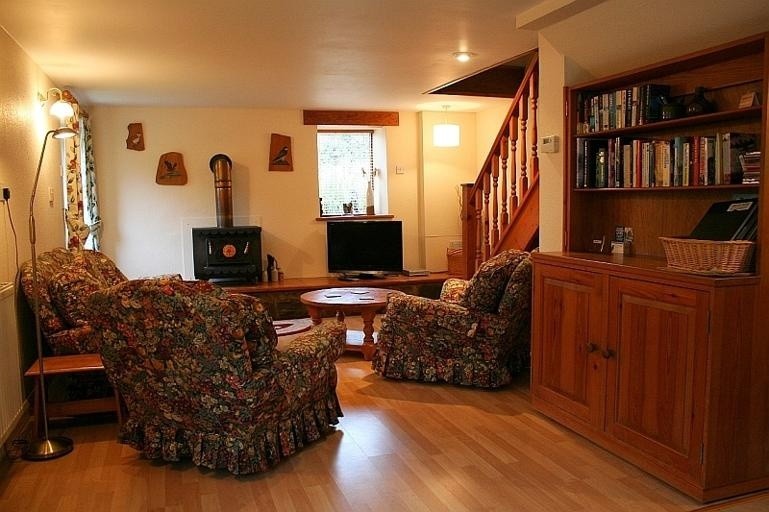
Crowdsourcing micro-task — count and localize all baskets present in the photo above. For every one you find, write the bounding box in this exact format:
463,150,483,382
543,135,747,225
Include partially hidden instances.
660,233,756,274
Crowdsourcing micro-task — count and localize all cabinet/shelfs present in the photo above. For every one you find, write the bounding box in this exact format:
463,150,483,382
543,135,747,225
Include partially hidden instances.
525,247,768,502
212,272,465,321
560,29,768,274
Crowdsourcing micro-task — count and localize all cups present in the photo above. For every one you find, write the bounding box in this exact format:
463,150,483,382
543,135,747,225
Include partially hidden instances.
658,103,681,120
343,204,352,214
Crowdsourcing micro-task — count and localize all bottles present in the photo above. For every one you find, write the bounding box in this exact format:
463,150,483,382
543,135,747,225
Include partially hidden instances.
262,271,269,282
685,87,714,115
271,266,284,282
320,198,323,216
367,181,376,216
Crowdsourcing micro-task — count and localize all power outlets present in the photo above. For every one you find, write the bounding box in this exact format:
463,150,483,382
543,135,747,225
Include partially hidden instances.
0,183,8,201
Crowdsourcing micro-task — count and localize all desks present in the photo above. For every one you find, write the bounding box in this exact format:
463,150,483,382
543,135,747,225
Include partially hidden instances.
24,351,123,441
299,286,407,362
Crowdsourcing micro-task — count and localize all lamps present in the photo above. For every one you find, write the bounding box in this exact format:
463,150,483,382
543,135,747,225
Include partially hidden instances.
19,86,80,463
431,105,462,148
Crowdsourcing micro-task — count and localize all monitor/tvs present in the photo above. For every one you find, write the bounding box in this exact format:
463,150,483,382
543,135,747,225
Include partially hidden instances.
326,220,403,274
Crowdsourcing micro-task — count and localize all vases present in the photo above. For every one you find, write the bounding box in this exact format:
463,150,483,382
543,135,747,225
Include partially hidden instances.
366,181,375,215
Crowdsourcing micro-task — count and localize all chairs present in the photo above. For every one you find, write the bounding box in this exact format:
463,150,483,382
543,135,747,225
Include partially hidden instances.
83,273,348,481
368,243,539,392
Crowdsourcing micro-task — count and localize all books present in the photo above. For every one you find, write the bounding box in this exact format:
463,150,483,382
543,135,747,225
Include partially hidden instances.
572,80,762,192
689,198,758,242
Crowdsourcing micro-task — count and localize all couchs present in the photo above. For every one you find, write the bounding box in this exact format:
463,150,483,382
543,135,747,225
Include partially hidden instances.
19,244,131,418
